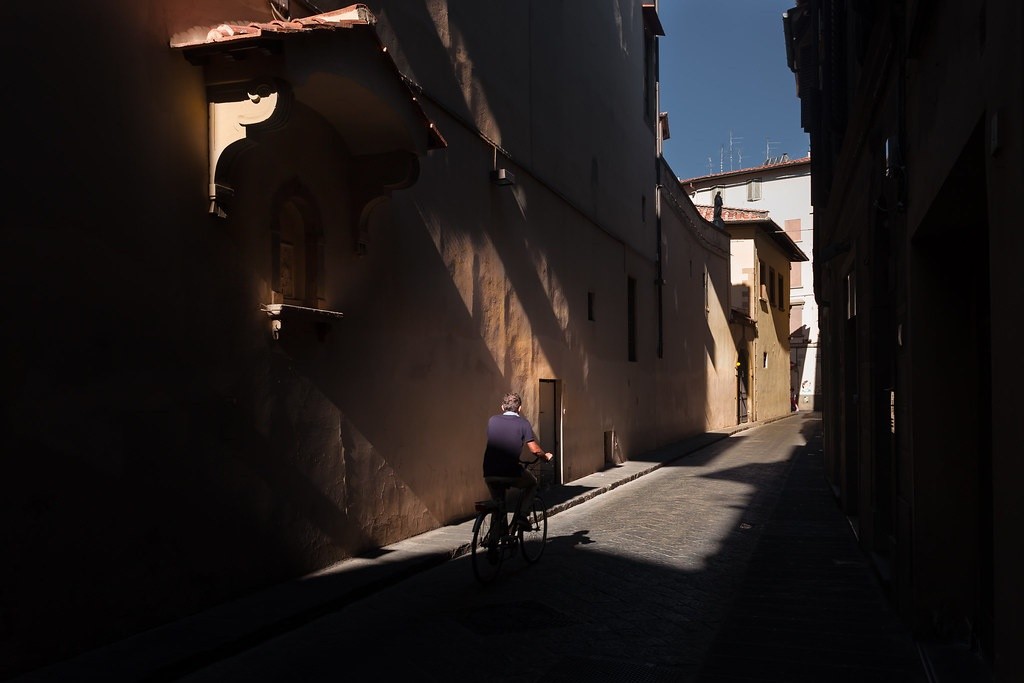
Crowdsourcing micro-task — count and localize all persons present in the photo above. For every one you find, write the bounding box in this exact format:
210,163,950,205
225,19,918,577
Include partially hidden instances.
483,392,552,564
791,387,797,412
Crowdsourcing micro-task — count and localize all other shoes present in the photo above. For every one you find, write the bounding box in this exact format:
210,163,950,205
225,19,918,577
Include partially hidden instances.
517,515,533,532
485,550,501,558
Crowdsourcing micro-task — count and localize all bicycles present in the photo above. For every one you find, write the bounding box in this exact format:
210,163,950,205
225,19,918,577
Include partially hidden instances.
472,450,559,583
